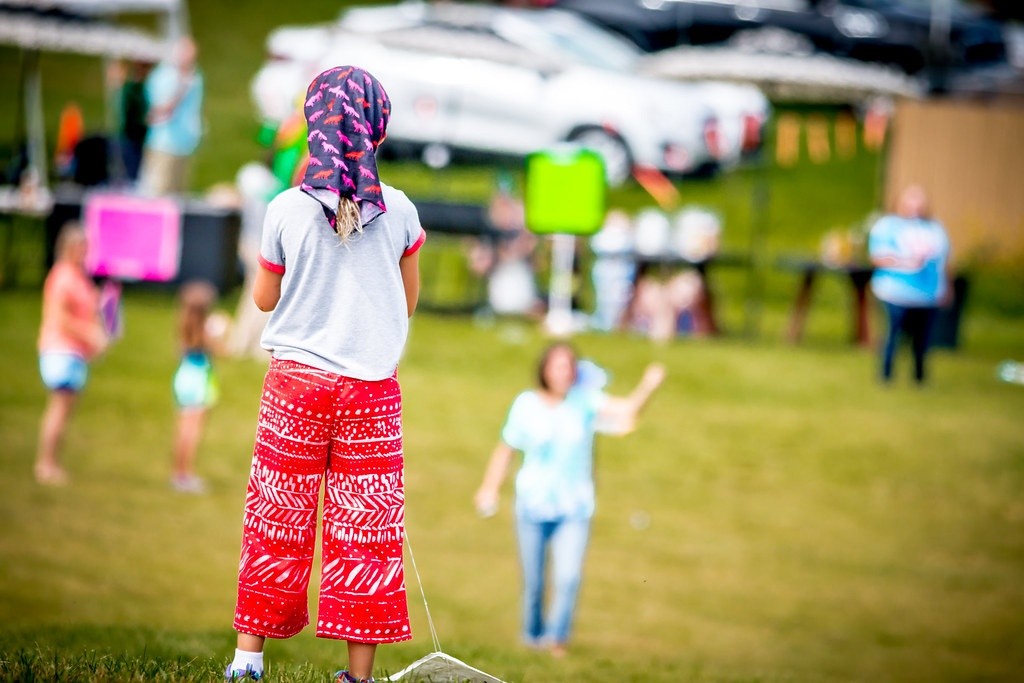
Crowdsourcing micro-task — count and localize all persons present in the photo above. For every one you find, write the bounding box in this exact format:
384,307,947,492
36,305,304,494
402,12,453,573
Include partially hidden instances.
216,64,428,683
50,88,87,181
100,56,135,184
133,28,209,201
861,178,952,388
163,276,225,495
226,130,311,368
472,336,668,655
453,188,722,336
123,61,159,179
30,220,113,491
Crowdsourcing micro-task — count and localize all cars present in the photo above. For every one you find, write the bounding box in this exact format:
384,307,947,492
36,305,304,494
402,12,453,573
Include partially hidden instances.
560,0,1016,108
250,3,773,199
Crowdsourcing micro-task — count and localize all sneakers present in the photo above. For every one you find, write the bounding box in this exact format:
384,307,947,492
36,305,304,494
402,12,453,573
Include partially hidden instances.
223,662,264,681
333,666,373,683
